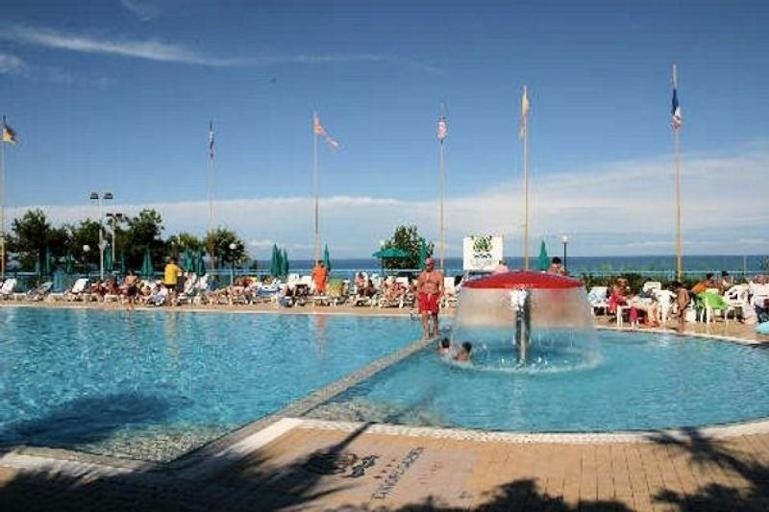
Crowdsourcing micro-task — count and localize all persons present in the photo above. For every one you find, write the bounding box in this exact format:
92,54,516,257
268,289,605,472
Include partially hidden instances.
279,272,419,308
452,341,472,363
416,258,445,340
435,337,452,355
548,257,769,332
71,269,259,311
23,279,45,304
311,260,328,293
0,278,6,288
163,257,182,296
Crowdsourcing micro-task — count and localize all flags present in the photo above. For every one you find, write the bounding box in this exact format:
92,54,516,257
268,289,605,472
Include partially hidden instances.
312,111,338,148
668,75,681,132
518,94,531,143
207,129,215,160
436,116,448,142
0,126,16,145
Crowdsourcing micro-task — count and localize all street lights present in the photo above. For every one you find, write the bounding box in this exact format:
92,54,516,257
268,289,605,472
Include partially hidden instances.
561,235,568,272
83,245,90,278
229,243,236,285
378,239,385,278
88,192,112,283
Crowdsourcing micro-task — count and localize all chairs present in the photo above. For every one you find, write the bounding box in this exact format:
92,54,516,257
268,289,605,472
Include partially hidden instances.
0,273,419,311
590,280,750,328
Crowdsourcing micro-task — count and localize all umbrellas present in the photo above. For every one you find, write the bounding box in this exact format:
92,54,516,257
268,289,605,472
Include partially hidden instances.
325,244,331,273
183,243,206,278
104,243,154,281
34,247,73,277
534,241,552,271
369,237,427,275
271,244,289,281
